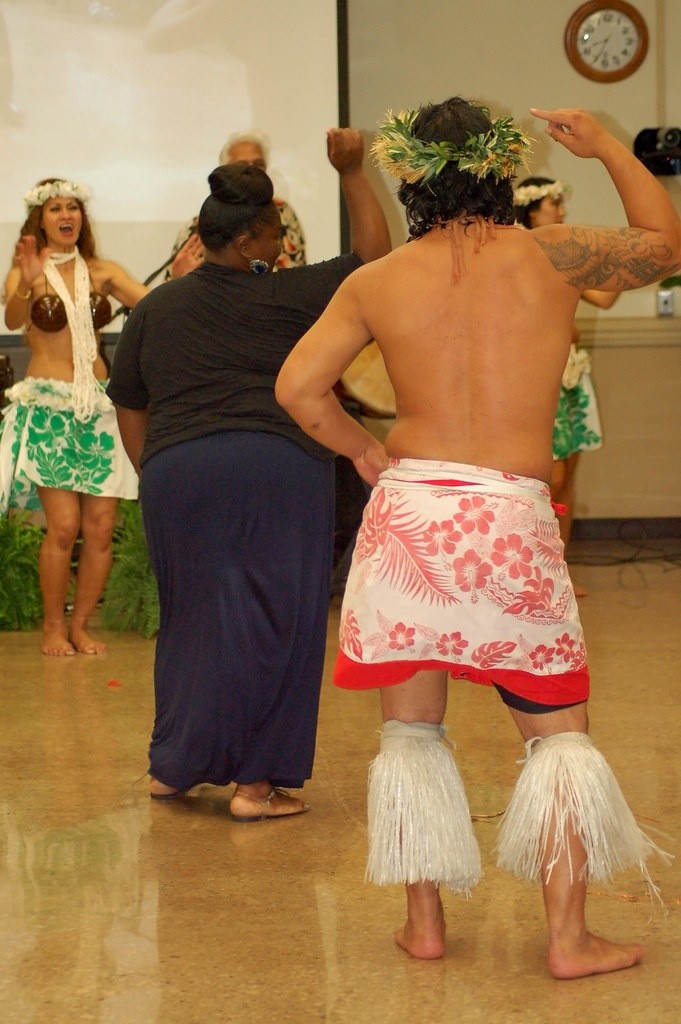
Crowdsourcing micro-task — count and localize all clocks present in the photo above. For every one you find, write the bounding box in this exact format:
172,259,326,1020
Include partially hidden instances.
564,0,650,83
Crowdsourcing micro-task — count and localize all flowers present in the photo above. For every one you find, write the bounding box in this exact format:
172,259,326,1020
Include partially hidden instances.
24,180,89,214
369,99,540,196
513,180,572,207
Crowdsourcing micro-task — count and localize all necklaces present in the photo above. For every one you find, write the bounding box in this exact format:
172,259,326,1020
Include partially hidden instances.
410,214,505,282
42,244,106,423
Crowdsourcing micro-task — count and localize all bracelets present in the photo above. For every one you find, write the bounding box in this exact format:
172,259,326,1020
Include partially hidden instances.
15,288,31,300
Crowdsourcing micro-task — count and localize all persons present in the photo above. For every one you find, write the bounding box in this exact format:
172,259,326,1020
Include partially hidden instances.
274,96,681,979
163,126,306,285
105,126,393,821
512,176,623,596
0,178,151,657
